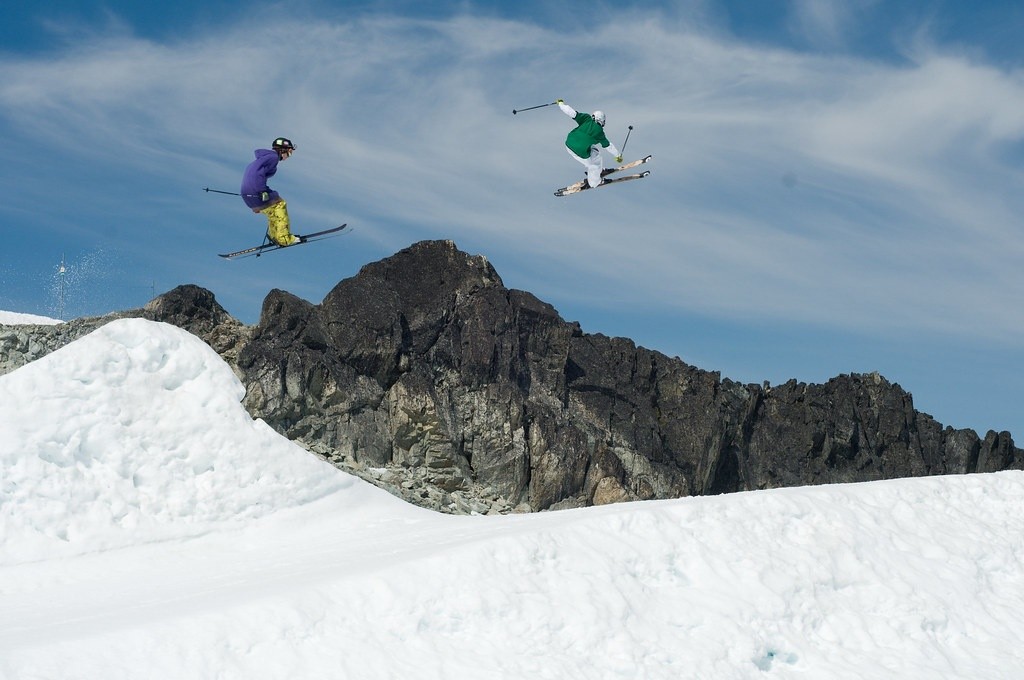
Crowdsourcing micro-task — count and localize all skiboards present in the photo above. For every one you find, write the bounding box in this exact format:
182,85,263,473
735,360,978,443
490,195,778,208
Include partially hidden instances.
226,228,355,270
216,222,348,258
557,154,654,193
552,170,651,197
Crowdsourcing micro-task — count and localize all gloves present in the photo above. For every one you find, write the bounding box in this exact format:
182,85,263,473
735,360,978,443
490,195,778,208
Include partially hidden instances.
260,191,269,201
555,98,563,105
614,154,623,162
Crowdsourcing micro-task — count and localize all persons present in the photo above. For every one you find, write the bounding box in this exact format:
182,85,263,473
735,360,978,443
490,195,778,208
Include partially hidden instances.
240,137,308,250
556,98,623,189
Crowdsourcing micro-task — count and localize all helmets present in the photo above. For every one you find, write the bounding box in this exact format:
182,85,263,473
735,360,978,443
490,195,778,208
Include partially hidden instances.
592,110,605,127
272,138,295,153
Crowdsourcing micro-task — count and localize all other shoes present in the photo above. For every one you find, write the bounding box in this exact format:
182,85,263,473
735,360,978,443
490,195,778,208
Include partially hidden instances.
592,178,612,186
284,235,306,245
601,168,615,176
